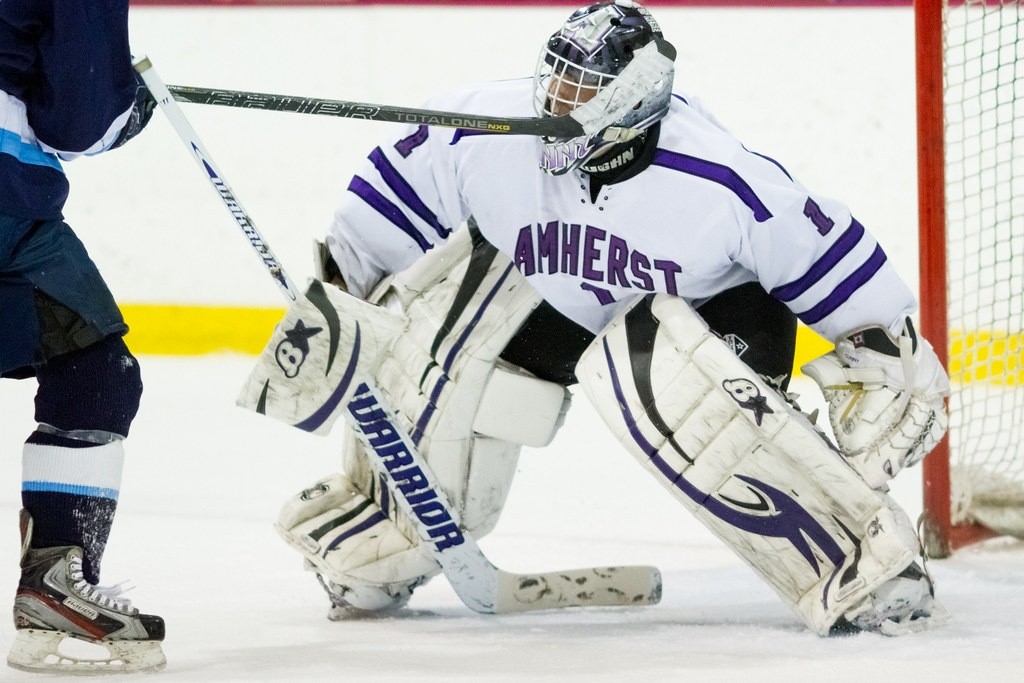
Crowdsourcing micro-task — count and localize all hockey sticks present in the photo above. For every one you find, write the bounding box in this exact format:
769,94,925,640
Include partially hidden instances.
167,39,681,138
129,52,663,617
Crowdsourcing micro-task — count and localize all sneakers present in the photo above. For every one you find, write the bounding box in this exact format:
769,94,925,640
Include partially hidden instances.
7,546,166,652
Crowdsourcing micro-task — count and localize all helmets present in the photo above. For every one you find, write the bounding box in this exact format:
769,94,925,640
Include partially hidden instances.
533,0,678,176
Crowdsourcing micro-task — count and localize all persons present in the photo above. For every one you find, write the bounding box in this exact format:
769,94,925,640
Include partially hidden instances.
0,0,165,676
233,0,953,636
